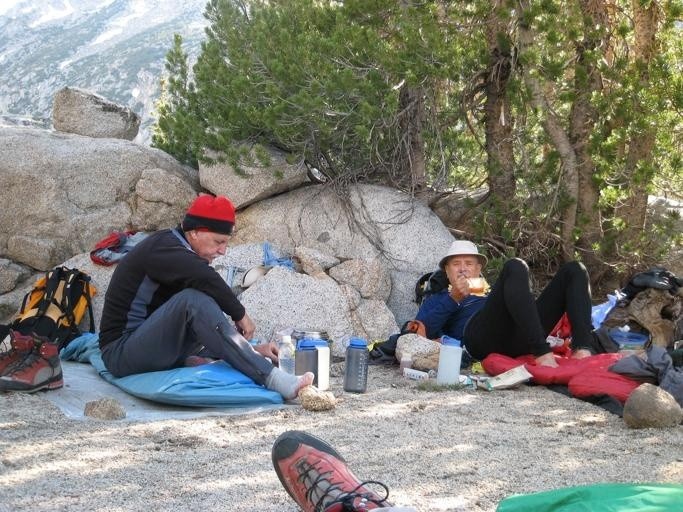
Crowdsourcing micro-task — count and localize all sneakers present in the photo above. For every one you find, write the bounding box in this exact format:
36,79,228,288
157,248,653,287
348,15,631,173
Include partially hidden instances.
0,331,63,395
0,329,32,373
269,429,392,512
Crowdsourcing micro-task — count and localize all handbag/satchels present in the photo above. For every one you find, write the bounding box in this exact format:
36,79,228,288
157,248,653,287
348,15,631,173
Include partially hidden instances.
415,271,451,303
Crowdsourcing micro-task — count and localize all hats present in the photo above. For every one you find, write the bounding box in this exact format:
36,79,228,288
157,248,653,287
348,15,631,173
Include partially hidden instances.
439,240,488,272
183,194,236,235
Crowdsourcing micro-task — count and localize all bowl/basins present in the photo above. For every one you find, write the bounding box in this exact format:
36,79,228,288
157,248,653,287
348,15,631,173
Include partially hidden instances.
609,332,649,348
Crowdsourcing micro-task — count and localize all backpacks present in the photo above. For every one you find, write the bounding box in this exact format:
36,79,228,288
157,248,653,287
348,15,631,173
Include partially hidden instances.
14,267,95,330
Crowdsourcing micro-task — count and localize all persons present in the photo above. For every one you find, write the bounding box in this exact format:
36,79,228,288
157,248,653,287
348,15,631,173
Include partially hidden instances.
407,239,595,371
96,194,317,404
266,426,415,512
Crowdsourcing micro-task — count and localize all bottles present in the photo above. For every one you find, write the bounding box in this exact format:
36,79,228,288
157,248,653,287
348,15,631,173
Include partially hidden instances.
400,353,412,375
437,335,464,388
341,338,370,394
295,340,319,391
278,335,295,376
313,340,330,391
